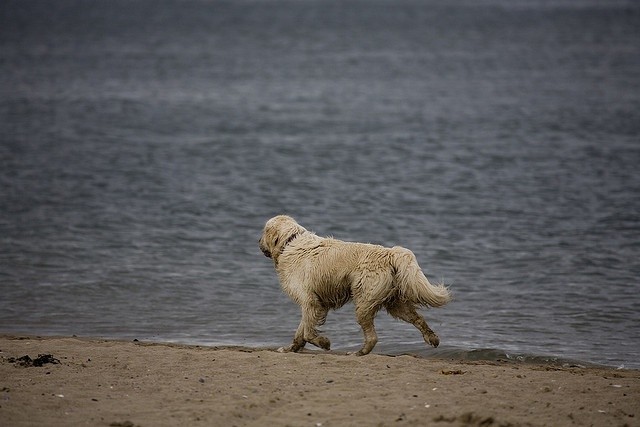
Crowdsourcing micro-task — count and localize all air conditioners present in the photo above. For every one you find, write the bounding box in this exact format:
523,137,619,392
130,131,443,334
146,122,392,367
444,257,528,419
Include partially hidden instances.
258,216,449,356
